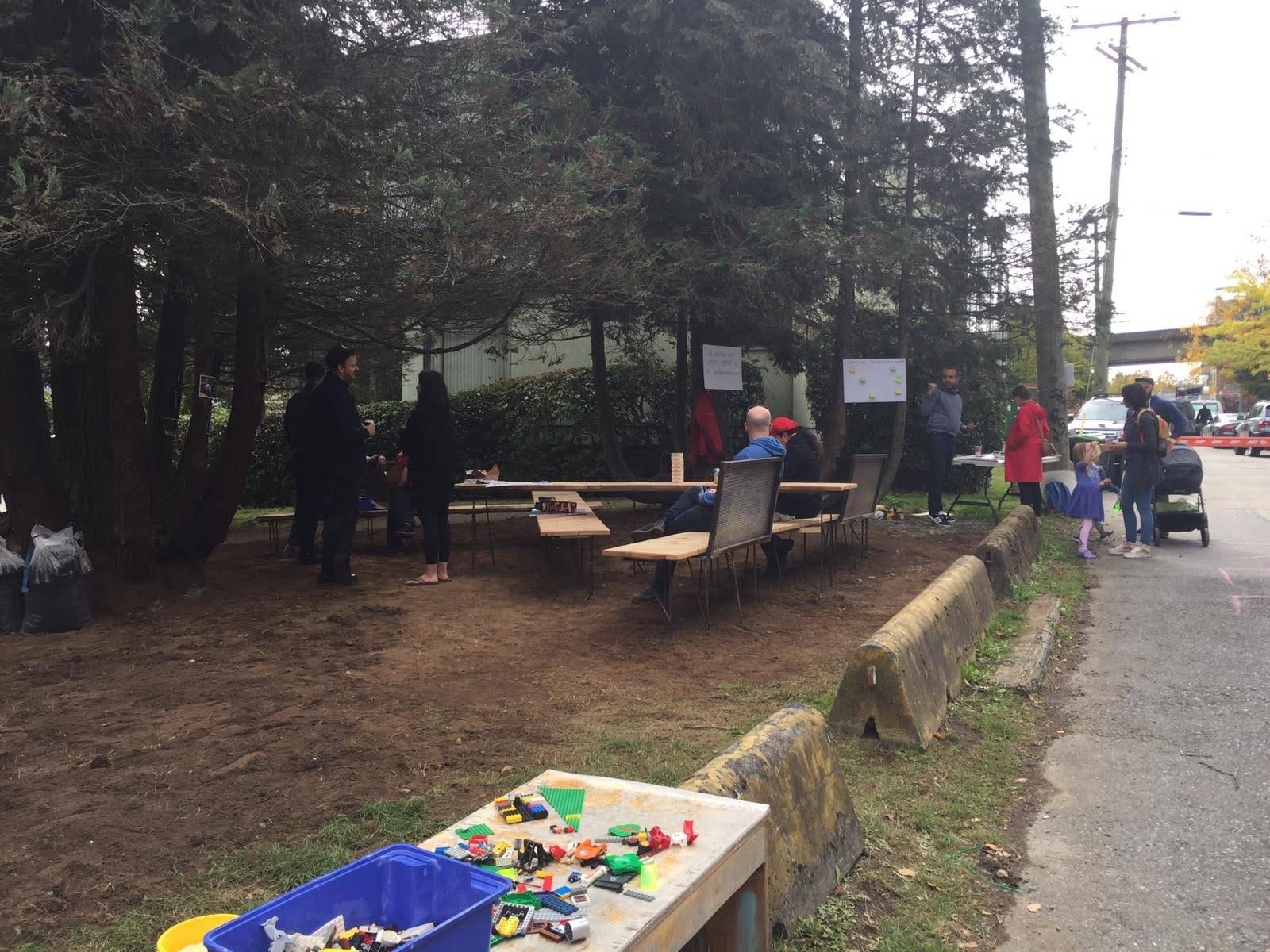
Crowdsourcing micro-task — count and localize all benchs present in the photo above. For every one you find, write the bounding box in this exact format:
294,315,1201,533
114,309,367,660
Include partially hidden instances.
256,501,840,630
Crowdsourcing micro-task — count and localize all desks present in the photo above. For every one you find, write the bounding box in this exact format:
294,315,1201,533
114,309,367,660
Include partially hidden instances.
453,482,859,577
416,767,773,952
947,454,1019,526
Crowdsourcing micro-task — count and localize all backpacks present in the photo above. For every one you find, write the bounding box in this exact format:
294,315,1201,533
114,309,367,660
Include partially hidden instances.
1135,407,1175,457
1043,480,1073,515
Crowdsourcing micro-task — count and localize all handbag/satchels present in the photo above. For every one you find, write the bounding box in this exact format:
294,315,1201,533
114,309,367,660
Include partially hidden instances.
1041,437,1056,456
19,523,94,637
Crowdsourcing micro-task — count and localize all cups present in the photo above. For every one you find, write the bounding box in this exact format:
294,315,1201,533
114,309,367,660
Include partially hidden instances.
1112,439,1118,442
363,419,374,426
974,445,982,457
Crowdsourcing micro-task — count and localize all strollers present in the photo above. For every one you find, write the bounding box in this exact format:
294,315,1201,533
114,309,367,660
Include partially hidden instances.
1151,443,1210,548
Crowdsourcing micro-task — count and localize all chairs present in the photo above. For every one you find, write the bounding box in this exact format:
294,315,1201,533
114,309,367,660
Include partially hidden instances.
689,456,784,636
804,454,890,593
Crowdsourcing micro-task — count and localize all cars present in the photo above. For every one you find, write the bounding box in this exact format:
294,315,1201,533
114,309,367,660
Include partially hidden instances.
1189,399,1250,450
1067,394,1129,463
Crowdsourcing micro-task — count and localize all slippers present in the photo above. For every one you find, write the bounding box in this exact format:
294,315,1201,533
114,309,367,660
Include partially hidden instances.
403,576,452,585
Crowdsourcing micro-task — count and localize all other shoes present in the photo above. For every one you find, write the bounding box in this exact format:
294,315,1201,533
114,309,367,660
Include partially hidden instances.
762,536,794,574
317,572,357,584
297,549,321,564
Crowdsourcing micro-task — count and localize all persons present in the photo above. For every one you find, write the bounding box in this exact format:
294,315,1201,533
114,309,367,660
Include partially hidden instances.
1197,404,1212,421
1132,378,1188,539
282,362,326,564
1072,444,1122,543
629,404,786,603
305,344,419,586
1066,440,1112,560
398,370,455,585
1108,383,1165,561
755,417,822,576
919,364,977,528
1171,387,1197,435
1003,385,1049,517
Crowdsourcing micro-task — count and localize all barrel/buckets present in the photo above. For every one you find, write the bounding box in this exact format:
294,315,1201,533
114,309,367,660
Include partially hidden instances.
157,913,240,952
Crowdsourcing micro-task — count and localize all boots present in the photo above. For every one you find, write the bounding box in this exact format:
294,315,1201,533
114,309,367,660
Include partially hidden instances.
630,513,670,541
632,582,666,604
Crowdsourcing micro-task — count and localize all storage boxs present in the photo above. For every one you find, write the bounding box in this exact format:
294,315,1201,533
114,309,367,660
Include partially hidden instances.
203,843,513,952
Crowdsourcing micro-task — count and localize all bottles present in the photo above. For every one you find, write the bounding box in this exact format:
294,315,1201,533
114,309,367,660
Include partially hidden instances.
359,497,372,511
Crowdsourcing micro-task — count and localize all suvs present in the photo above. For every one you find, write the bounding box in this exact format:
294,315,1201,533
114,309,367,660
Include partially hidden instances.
1235,400,1270,458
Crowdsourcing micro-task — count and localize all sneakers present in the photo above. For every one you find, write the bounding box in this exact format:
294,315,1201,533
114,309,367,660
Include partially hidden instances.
928,510,955,527
1123,541,1151,558
1077,545,1097,559
1108,540,1137,555
383,546,407,555
1099,530,1114,540
394,521,417,538
1072,525,1092,542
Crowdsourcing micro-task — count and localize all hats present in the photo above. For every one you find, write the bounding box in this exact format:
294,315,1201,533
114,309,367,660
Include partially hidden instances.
327,345,355,369
305,361,331,381
1177,388,1185,396
770,417,801,433
1135,375,1153,387
